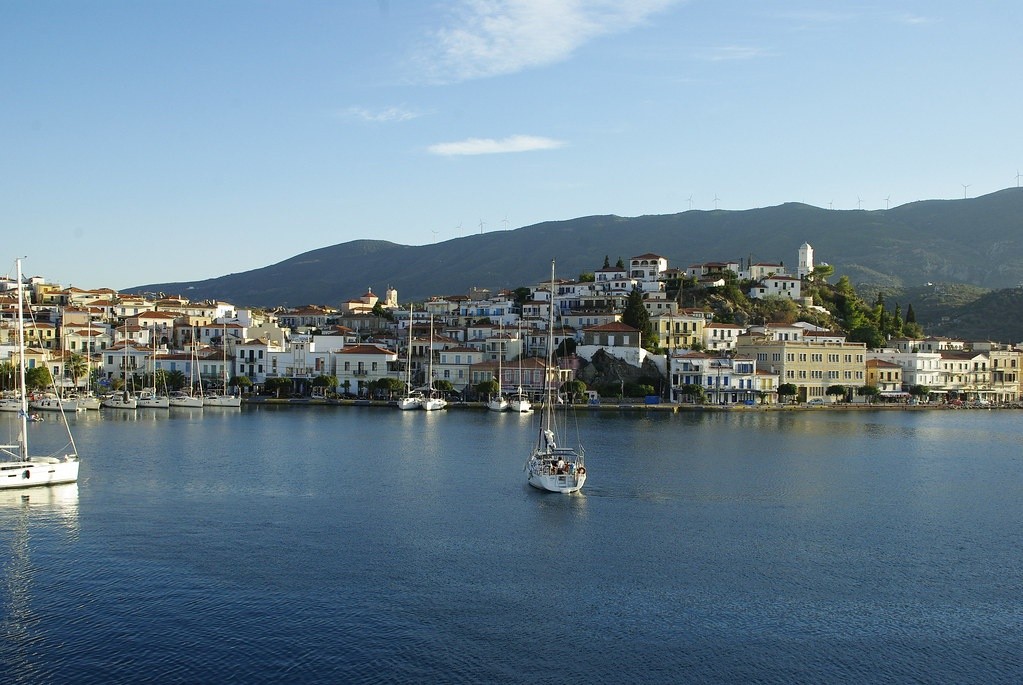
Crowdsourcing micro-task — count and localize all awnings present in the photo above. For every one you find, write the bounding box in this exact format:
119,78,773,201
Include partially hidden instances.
881,392,910,397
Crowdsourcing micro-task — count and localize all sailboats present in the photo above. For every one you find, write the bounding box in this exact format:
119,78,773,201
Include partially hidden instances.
0,306,243,413
521,258,586,494
396,304,423,410
511,316,532,413
0,254,81,488
422,312,447,411
486,317,509,412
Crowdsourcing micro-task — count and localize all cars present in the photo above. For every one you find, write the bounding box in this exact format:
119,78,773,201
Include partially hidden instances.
948,399,963,406
975,398,991,406
808,397,824,405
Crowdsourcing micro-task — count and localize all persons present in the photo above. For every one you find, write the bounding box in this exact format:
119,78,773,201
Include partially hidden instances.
556,457,565,479
30,413,39,420
565,462,570,473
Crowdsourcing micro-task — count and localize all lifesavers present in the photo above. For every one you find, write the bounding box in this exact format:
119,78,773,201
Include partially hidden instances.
579,467,586,474
